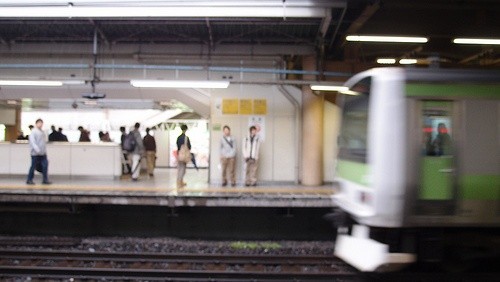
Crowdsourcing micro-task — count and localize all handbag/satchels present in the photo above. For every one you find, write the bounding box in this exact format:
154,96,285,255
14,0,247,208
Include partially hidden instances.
178,135,192,163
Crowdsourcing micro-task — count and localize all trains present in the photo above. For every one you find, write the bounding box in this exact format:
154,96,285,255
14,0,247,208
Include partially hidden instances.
332,68,500,272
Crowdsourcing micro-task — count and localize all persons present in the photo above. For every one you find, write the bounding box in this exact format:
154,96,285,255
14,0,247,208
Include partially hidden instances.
26,125,35,139
18,123,111,141
219,125,237,186
119,127,131,174
242,125,259,186
176,125,191,185
26,118,51,186
143,128,156,176
433,122,450,155
129,123,145,179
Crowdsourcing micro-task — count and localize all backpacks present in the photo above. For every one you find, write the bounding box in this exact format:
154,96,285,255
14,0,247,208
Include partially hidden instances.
124,132,136,152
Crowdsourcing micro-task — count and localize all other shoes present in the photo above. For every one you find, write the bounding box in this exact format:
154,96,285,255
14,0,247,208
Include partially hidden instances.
27,181,34,184
43,181,50,184
